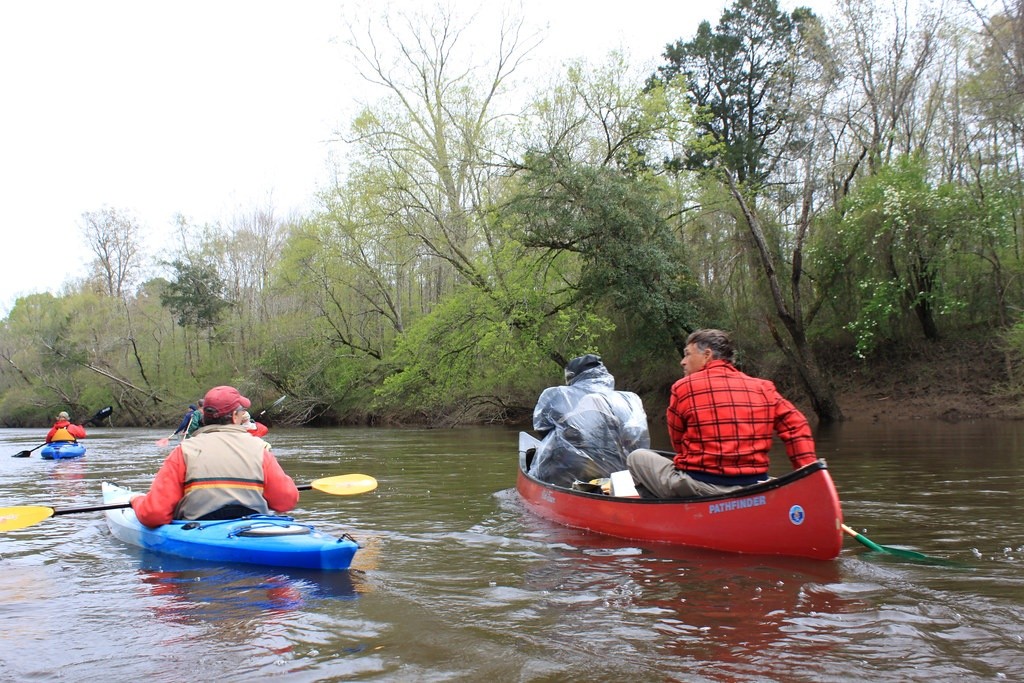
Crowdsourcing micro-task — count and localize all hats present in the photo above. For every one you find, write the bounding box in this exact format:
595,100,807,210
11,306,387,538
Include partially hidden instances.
198,399,204,405
55,411,71,421
188,405,196,411
203,386,251,418
564,354,604,385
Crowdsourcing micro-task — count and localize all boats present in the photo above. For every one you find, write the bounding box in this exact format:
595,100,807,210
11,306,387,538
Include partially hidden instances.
101,480,360,571
40,443,87,459
249,422,270,439
514,428,844,562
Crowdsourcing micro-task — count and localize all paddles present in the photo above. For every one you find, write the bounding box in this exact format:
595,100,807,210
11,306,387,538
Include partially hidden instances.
158,431,177,444
842,523,923,560
2,472,379,532
251,395,286,421
13,405,113,457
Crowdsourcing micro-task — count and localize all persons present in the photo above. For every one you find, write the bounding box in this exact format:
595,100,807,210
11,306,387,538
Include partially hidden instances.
527,353,650,495
173,400,267,439
130,386,299,526
47,408,85,446
625,330,817,502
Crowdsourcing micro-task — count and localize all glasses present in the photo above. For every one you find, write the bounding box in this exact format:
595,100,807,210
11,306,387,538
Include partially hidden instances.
234,407,250,415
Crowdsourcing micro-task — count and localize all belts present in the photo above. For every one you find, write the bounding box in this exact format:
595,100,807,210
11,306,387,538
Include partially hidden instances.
686,470,769,486
